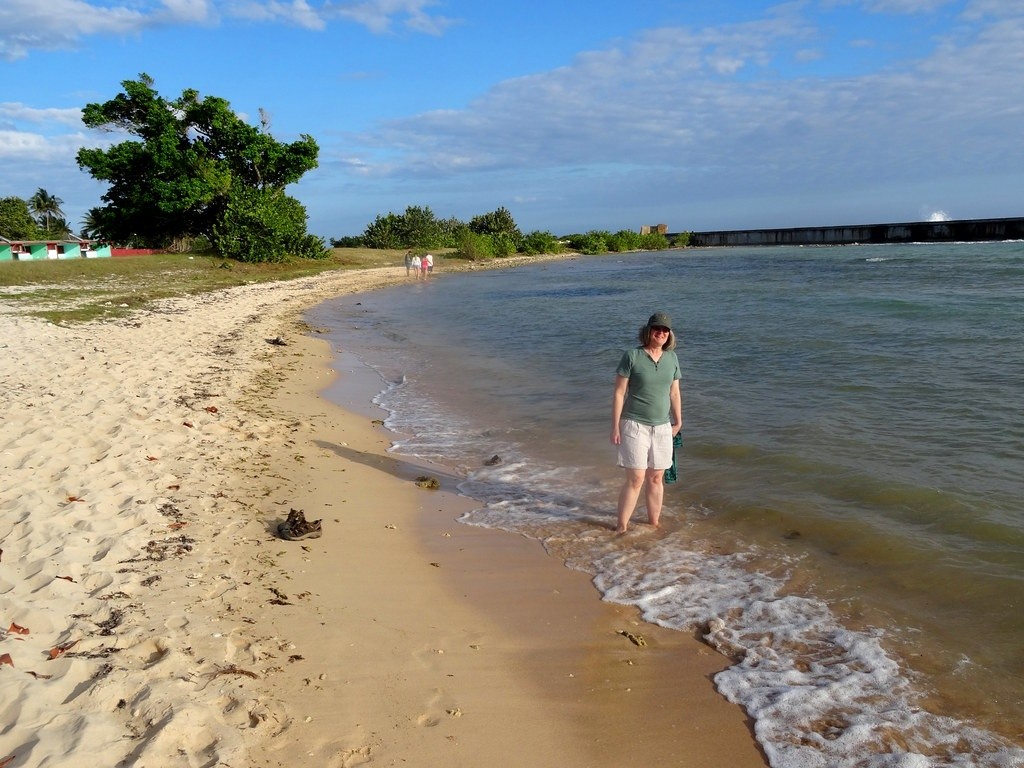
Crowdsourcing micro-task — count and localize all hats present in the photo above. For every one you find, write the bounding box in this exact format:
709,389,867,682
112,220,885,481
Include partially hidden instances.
648,313,672,330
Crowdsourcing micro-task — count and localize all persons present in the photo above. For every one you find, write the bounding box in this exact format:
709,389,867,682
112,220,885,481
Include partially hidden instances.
404,251,434,280
610,311,682,534
